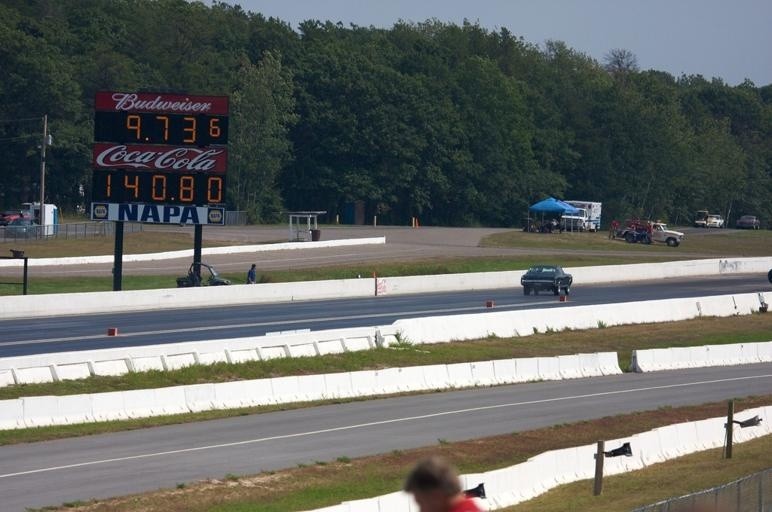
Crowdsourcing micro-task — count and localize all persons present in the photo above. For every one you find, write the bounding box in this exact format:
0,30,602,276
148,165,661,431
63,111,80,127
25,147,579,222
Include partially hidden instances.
608,216,619,240
403,456,483,511
246,263,257,283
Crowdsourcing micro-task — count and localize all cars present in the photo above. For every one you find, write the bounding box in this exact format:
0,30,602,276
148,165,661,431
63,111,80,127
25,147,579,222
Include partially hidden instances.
693,209,762,231
521,264,576,297
1,199,58,239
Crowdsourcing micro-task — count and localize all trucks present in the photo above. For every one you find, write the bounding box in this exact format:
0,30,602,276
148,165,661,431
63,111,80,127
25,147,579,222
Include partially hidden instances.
558,200,602,233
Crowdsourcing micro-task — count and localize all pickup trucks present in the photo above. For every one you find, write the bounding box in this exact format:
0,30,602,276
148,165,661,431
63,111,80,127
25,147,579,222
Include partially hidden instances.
616,218,686,248
